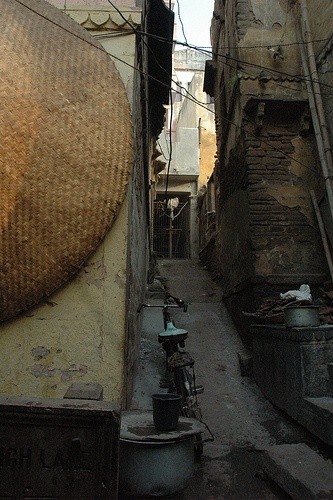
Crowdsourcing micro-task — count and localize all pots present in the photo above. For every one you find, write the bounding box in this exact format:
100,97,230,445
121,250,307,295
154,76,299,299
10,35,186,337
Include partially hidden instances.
283,306,320,327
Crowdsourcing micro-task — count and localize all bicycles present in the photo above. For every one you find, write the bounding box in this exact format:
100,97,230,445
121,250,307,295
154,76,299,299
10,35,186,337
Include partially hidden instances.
136,303,216,454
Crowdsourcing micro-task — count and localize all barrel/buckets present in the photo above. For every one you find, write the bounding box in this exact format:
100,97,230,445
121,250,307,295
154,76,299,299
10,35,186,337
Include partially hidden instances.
151,393,184,430
